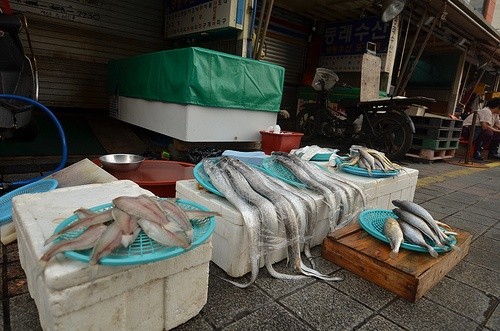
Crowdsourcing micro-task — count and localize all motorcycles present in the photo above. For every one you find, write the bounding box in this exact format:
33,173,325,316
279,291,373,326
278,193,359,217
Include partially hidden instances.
296,68,436,160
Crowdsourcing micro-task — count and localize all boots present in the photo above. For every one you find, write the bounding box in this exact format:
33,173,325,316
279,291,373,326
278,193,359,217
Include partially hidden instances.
473,139,484,161
487,141,500,160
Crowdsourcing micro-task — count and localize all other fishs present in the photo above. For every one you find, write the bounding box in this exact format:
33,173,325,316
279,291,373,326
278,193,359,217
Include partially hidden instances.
202,144,462,289
31,194,224,279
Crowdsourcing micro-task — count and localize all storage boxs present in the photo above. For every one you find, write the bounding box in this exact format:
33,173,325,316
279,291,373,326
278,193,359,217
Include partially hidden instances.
13,179,213,331
173,164,419,277
109,47,286,144
321,218,473,302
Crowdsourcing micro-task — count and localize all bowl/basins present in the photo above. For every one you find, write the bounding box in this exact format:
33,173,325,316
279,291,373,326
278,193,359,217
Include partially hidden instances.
98,154,146,172
92,159,198,198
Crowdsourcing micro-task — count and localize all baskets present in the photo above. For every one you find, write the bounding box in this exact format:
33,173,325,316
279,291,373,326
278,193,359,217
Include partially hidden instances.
312,67,339,90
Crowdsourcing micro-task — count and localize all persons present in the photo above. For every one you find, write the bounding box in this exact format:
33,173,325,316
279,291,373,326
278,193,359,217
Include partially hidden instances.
461,97,500,162
